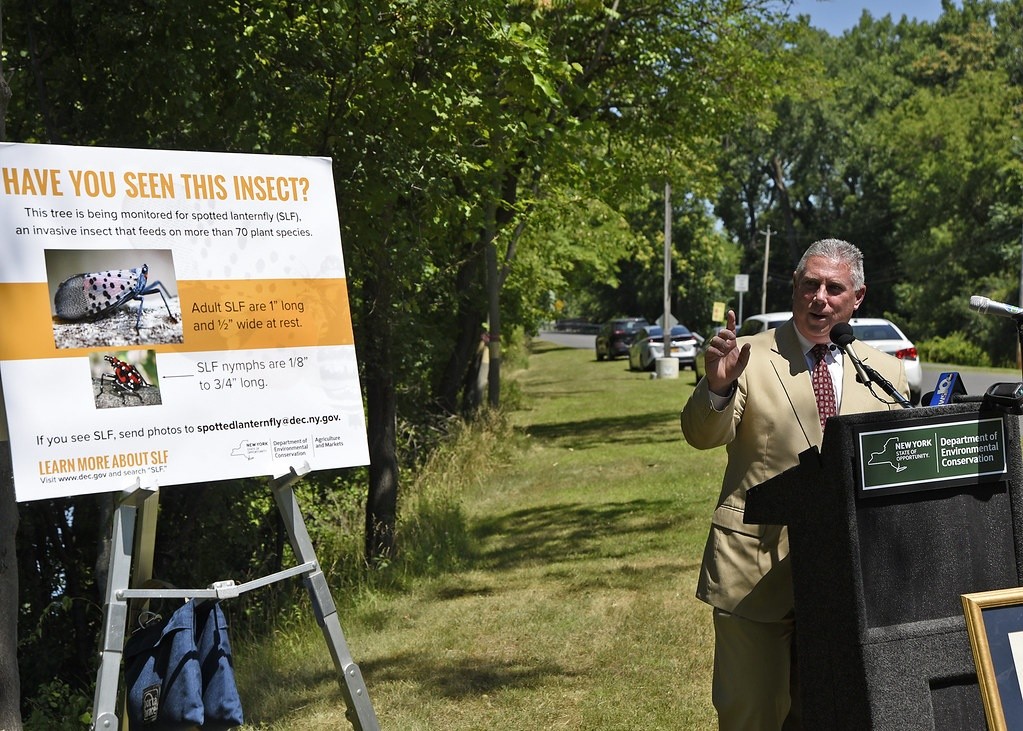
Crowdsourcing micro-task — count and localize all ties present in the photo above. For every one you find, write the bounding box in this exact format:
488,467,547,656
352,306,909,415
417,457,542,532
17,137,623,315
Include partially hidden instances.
810,344,838,431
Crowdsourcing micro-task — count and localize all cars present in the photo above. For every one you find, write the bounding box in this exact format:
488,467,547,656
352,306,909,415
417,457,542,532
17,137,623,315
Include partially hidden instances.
735,311,794,337
848,317,922,405
628,324,699,370
693,326,739,381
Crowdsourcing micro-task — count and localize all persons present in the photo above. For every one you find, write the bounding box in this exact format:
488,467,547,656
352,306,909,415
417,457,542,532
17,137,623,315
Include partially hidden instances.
680,238,911,731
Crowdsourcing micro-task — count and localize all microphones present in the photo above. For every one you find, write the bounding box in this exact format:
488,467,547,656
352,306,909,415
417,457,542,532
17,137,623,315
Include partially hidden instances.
921,373,1023,416
829,323,871,385
830,344,836,352
969,295,1023,320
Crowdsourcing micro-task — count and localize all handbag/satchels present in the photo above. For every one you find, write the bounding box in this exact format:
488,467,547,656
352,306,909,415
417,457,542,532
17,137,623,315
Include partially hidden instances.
124,597,244,731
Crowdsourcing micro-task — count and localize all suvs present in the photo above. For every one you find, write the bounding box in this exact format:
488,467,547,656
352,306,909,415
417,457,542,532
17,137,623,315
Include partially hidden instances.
595,318,649,360
